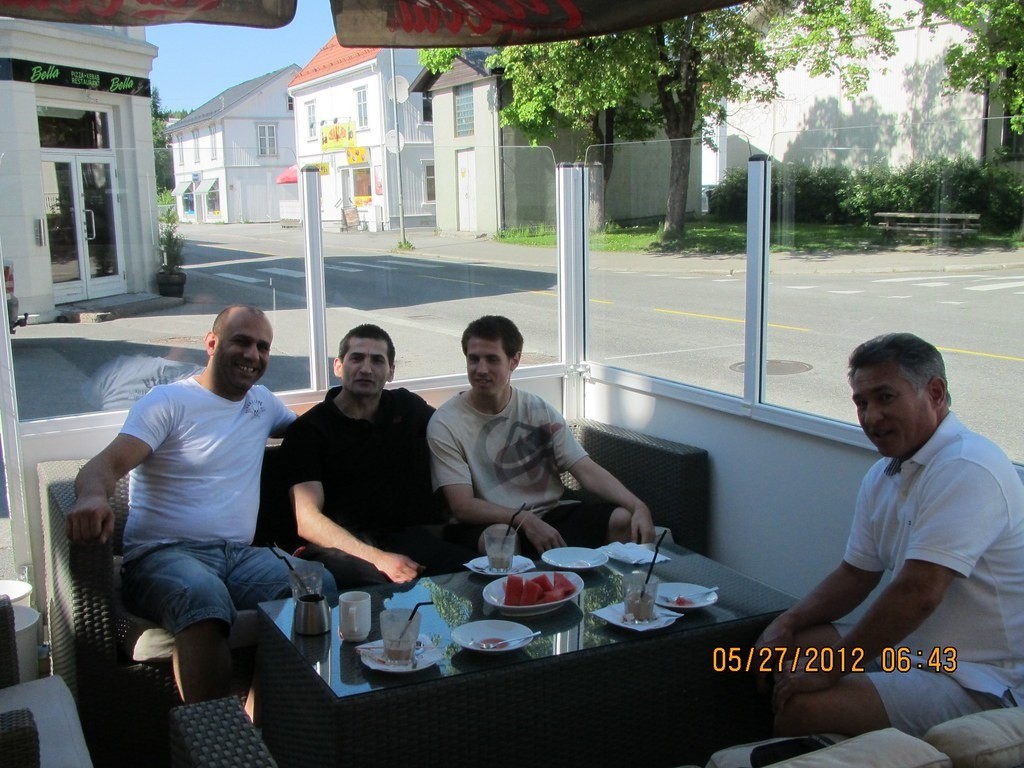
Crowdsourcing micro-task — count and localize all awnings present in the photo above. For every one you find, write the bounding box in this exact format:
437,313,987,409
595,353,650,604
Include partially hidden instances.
276,164,297,183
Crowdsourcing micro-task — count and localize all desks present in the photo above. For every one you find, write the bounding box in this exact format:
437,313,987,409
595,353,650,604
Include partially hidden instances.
874,211,981,246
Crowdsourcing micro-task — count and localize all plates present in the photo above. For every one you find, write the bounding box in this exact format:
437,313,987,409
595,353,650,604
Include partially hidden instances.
655,582,718,609
451,620,534,654
355,634,444,673
588,601,685,632
541,547,608,570
462,555,536,580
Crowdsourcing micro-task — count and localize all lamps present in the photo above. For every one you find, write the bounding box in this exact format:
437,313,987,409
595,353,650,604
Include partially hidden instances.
310,117,351,128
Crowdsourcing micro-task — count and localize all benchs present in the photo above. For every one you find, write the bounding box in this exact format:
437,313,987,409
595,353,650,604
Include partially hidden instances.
35,418,706,768
168,697,1024,768
879,222,980,233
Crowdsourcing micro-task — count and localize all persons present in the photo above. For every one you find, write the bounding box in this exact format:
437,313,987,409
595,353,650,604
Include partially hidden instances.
749,332,1024,738
280,324,480,585
426,316,655,561
63,305,336,705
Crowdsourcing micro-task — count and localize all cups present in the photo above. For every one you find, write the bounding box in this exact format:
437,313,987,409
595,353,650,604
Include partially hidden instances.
292,593,332,635
338,590,371,642
288,561,324,610
622,573,658,624
379,608,422,662
484,524,517,573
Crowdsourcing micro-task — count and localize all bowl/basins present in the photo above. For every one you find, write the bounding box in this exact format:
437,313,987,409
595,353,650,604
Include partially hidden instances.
482,571,585,616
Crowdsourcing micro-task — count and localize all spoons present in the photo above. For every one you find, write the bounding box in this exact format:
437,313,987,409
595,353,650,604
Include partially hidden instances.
355,641,425,655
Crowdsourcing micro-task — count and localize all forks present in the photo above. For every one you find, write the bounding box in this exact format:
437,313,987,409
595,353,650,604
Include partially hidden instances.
474,631,542,649
659,587,719,602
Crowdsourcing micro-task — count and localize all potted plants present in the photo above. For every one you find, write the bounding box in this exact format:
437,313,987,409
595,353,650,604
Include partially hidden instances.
157,218,187,298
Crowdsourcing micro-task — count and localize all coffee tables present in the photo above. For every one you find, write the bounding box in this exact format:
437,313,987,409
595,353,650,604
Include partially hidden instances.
258,544,802,768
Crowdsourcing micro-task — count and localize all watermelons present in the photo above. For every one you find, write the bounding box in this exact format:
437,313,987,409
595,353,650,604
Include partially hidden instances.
503,573,575,606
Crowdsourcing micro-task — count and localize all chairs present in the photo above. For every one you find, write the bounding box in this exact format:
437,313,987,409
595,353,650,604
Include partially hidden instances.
0,594,94,768
